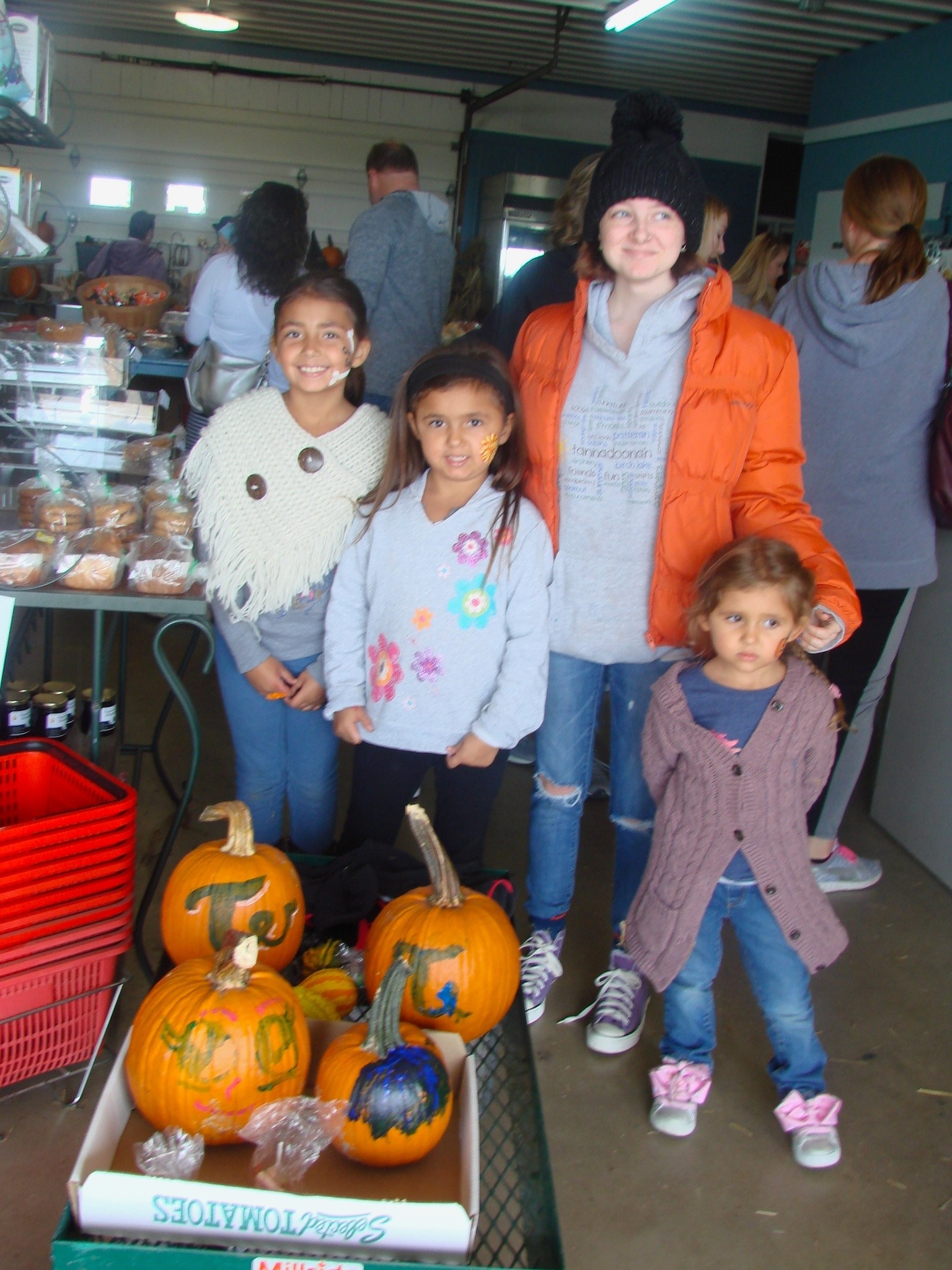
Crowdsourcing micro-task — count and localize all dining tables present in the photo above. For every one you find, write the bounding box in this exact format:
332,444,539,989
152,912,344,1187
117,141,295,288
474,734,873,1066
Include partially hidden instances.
1,584,216,1042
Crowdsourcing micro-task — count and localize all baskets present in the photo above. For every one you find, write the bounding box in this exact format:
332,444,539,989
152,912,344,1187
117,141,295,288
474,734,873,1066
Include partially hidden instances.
0,738,138,1088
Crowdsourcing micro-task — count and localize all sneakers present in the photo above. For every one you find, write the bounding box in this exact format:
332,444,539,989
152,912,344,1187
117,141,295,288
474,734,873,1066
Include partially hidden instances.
517,928,566,1025
555,951,650,1054
809,840,883,893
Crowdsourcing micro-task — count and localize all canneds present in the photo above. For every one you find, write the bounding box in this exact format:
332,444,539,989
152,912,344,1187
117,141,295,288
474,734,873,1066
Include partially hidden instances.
4,680,120,741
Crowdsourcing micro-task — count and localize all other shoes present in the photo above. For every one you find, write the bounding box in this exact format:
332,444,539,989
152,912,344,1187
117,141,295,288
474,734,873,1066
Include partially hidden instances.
585,754,612,800
648,1056,711,1136
774,1091,842,1168
507,738,536,763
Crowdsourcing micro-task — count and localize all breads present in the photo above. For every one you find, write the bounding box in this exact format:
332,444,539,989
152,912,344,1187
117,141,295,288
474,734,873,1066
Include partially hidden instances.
36,317,116,358
0,437,194,594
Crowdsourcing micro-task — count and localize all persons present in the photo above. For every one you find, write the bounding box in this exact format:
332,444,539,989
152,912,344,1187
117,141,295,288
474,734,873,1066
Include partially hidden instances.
475,153,605,361
86,207,169,283
724,231,791,317
342,140,457,412
619,531,858,1170
500,138,864,1056
179,266,401,850
325,342,557,962
766,153,952,899
178,182,324,458
217,221,235,255
702,194,728,270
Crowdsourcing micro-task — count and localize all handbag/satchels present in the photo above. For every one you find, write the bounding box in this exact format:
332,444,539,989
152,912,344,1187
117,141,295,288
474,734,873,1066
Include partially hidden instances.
184,336,269,417
930,280,952,529
95,239,117,278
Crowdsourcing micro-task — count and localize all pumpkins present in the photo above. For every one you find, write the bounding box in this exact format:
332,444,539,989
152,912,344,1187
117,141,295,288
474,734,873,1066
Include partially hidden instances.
125,802,522,1170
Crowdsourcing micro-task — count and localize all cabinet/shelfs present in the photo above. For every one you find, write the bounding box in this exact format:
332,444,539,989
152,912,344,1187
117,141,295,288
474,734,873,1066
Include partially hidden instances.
1,78,75,268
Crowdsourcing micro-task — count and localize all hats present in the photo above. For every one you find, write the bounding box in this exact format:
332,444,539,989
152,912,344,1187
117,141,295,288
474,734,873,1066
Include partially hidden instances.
212,216,236,244
582,91,706,261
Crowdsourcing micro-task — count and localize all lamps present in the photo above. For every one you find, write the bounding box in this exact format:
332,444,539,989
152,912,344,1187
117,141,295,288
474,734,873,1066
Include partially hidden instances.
175,0,239,32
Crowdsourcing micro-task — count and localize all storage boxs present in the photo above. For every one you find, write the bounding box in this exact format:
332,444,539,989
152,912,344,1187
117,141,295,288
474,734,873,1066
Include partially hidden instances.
50,1029,484,1269
